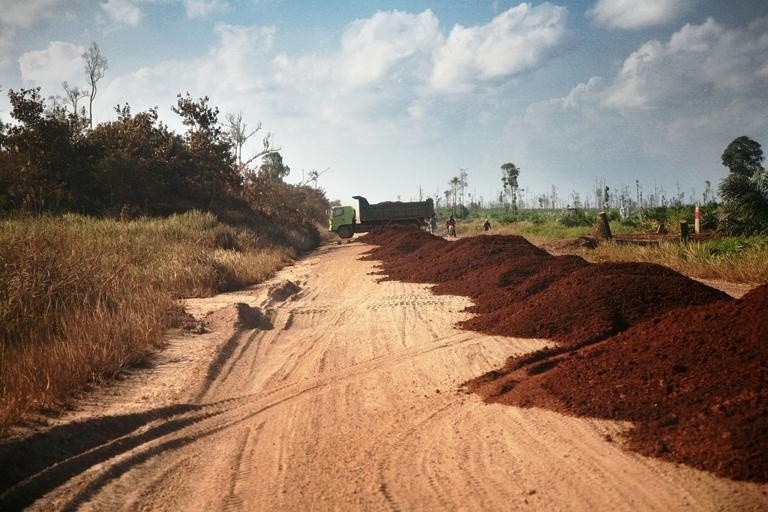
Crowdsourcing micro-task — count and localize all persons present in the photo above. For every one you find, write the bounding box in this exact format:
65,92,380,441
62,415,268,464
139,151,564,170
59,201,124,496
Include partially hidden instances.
446,215,457,238
423,217,431,231
430,213,437,234
483,218,491,231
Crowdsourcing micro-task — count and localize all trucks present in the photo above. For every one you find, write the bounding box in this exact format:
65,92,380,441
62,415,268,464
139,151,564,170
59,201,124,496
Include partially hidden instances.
328,196,435,240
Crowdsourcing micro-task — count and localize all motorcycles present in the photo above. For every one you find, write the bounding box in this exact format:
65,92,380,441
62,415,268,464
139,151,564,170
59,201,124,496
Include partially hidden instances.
448,224,454,236
